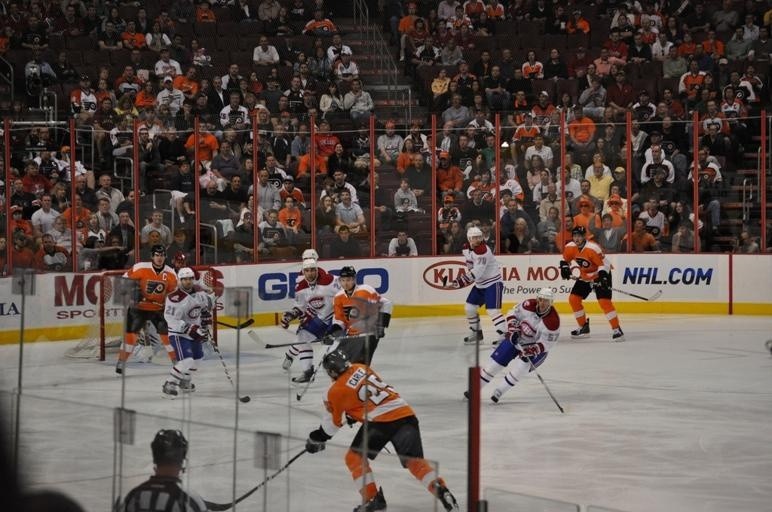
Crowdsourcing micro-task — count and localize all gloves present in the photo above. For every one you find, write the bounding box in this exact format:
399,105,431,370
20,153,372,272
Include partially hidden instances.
518,344,540,358
305,426,330,453
508,328,521,345
186,323,209,343
323,331,334,345
377,326,385,337
281,309,297,329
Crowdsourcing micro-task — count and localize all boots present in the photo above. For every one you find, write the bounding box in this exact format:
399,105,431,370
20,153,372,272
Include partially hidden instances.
435,487,461,512
464,329,484,342
353,487,386,512
281,353,316,382
611,326,624,339
570,318,589,335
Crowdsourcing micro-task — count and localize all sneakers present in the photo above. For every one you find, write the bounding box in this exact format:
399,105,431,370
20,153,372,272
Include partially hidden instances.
463,390,469,399
116,359,126,373
492,390,501,403
163,380,197,395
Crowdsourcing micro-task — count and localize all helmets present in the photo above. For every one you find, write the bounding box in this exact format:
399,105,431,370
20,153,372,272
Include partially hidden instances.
302,249,319,284
151,244,167,266
150,428,190,477
559,260,572,280
322,349,350,381
466,226,483,248
339,266,357,293
595,270,608,292
572,226,587,246
178,267,196,290
171,249,187,266
453,275,472,289
536,288,555,315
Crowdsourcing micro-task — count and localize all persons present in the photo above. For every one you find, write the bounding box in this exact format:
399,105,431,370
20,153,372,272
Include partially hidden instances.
115,244,177,374
279,258,341,383
1,2,772,286
463,288,561,403
114,428,215,512
443,227,508,349
306,349,462,511
161,267,225,400
328,267,393,369
563,226,626,342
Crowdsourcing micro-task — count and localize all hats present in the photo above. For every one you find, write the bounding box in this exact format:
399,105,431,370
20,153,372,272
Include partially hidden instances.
284,175,295,182
607,193,622,208
577,195,592,209
439,151,449,161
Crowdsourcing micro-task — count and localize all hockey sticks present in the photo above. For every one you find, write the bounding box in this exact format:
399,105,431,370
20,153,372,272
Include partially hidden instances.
442,276,458,291
204,447,306,511
569,275,663,302
207,328,252,403
293,335,335,401
517,342,567,413
248,330,331,346
